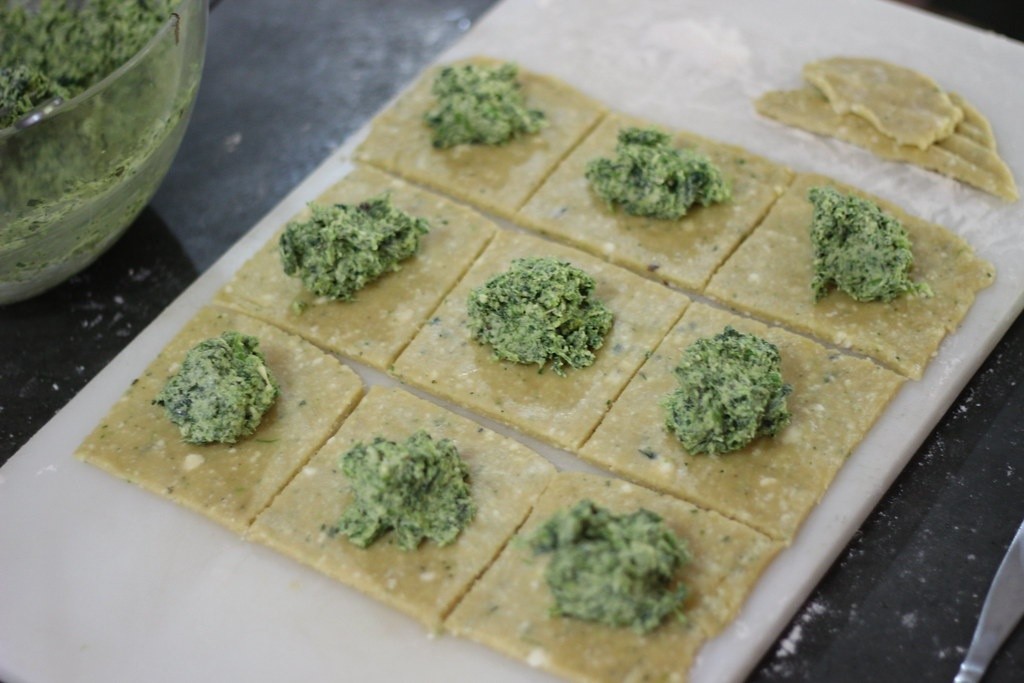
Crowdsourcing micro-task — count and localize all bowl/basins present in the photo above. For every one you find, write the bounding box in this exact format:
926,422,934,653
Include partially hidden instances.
0,0,208,304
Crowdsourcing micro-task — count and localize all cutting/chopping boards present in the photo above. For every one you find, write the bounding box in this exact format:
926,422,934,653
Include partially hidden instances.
0,0,1024,683
